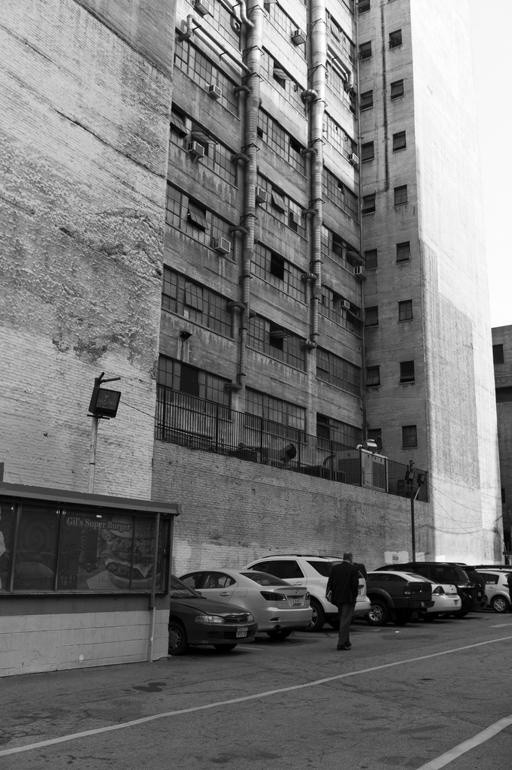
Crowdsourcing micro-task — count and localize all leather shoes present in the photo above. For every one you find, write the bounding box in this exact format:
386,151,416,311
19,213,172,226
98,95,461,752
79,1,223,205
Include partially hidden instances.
338,646,350,650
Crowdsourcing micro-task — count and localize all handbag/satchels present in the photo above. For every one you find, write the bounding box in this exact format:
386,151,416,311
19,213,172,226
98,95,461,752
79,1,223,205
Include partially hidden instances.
327,590,331,602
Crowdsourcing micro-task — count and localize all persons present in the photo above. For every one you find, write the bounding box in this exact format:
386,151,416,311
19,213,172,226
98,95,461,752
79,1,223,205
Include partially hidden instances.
325,551,360,651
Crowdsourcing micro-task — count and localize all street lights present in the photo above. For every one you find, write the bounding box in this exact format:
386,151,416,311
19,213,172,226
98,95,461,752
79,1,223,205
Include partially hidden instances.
406,460,427,563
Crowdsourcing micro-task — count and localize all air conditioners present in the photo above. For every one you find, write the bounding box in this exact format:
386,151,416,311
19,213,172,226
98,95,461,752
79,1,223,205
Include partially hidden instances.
355,265,367,280
213,236,232,255
342,299,351,310
188,141,206,160
194,0,211,16
349,153,360,166
346,80,358,96
210,84,223,100
334,447,389,492
292,28,307,45
255,186,267,204
